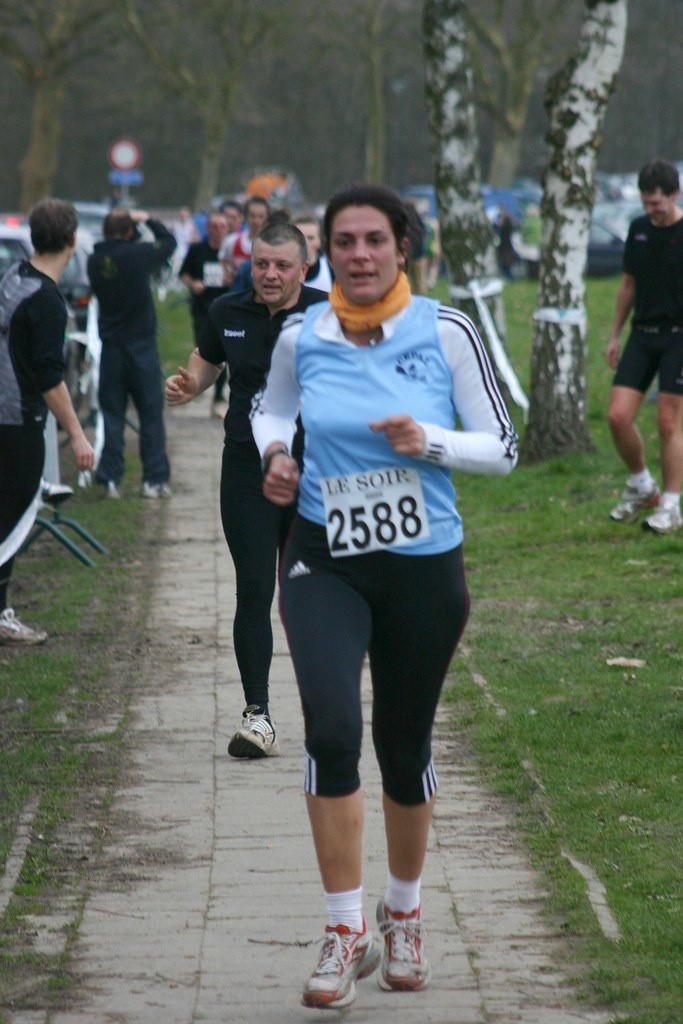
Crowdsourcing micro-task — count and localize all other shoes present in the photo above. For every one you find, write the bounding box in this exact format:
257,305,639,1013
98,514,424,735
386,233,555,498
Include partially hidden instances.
211,399,228,420
140,479,173,498
100,479,119,501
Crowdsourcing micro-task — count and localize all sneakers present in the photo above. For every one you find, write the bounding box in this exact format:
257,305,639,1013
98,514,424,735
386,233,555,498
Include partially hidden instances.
376,893,432,992
0,608,49,646
299,915,382,1010
228,712,281,758
641,498,683,535
609,482,661,524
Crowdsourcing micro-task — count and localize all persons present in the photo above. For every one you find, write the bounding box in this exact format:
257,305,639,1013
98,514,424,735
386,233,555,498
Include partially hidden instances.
177,202,332,418
247,182,518,1010
86,207,177,497
0,199,93,648
401,191,516,294
606,159,682,532
164,223,329,758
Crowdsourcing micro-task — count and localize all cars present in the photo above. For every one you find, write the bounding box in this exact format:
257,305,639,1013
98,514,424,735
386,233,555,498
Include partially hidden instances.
0,197,106,328
407,171,645,281
